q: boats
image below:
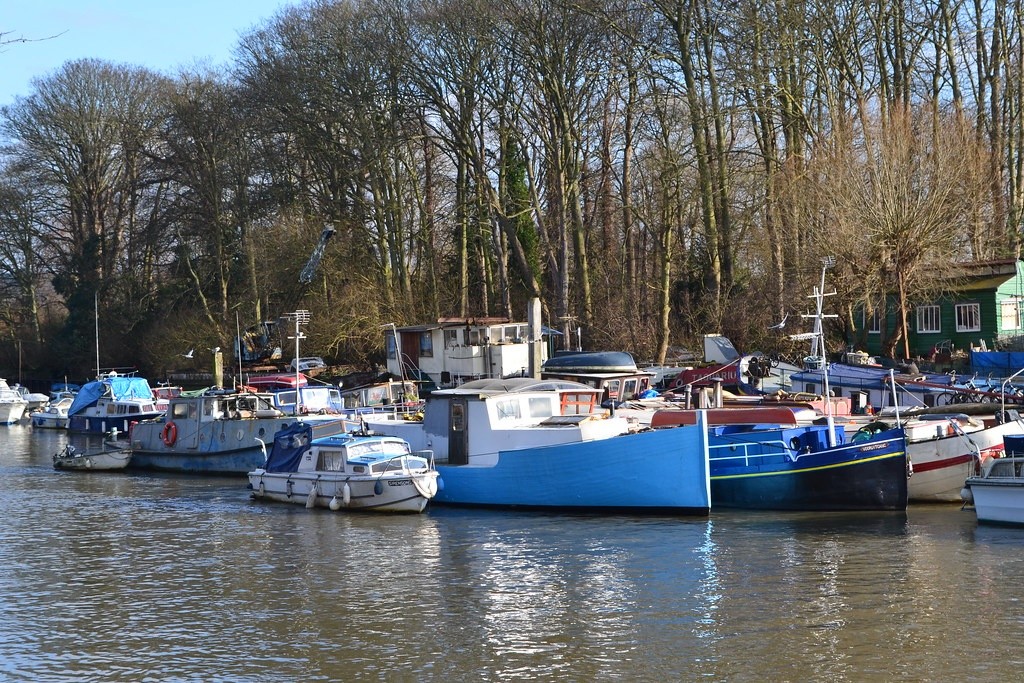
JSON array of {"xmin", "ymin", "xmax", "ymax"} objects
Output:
[{"xmin": 0, "ymin": 257, "xmax": 1023, "ymax": 531}]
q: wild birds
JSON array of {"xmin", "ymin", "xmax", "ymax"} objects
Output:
[
  {"xmin": 207, "ymin": 347, "xmax": 221, "ymax": 353},
  {"xmin": 180, "ymin": 350, "xmax": 195, "ymax": 358}
]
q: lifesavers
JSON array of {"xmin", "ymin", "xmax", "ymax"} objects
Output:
[{"xmin": 163, "ymin": 422, "xmax": 176, "ymax": 446}]
